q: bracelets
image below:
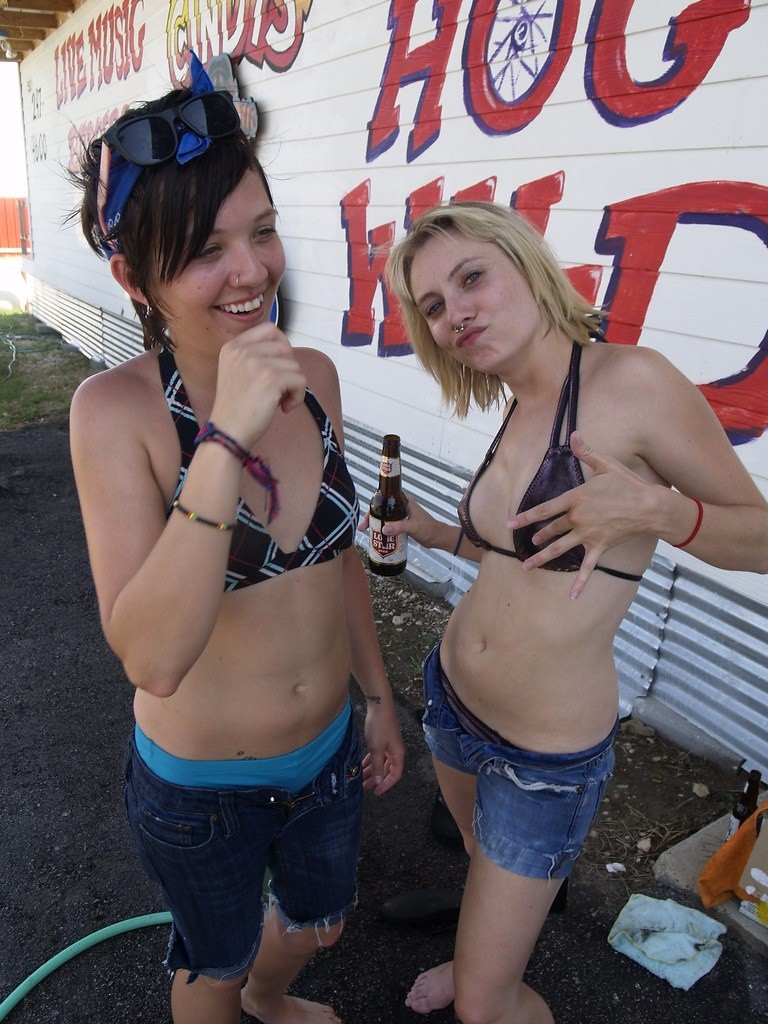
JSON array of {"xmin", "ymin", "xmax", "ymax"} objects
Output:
[
  {"xmin": 674, "ymin": 498, "xmax": 704, "ymax": 549},
  {"xmin": 173, "ymin": 502, "xmax": 237, "ymax": 530},
  {"xmin": 195, "ymin": 422, "xmax": 281, "ymax": 525}
]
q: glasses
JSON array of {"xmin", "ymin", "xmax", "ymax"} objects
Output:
[{"xmin": 98, "ymin": 89, "xmax": 241, "ymax": 252}]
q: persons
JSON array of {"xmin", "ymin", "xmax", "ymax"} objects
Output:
[
  {"xmin": 347, "ymin": 200, "xmax": 768, "ymax": 1024},
  {"xmin": 68, "ymin": 88, "xmax": 400, "ymax": 1024}
]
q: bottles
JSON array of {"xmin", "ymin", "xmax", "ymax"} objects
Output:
[
  {"xmin": 368, "ymin": 434, "xmax": 410, "ymax": 577},
  {"xmin": 725, "ymin": 770, "xmax": 761, "ymax": 842}
]
